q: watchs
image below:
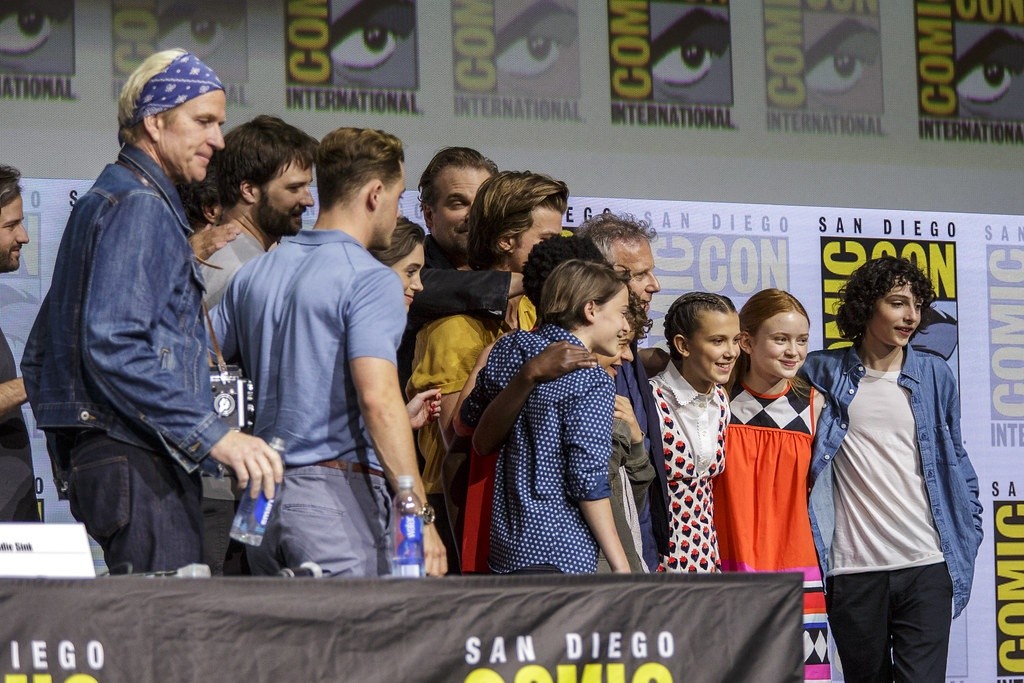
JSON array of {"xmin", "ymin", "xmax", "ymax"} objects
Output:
[{"xmin": 422, "ymin": 503, "xmax": 435, "ymax": 525}]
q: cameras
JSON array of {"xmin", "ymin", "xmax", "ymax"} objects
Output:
[{"xmin": 210, "ymin": 365, "xmax": 255, "ymax": 432}]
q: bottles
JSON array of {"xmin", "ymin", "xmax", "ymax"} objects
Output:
[
  {"xmin": 391, "ymin": 475, "xmax": 426, "ymax": 578},
  {"xmin": 230, "ymin": 436, "xmax": 285, "ymax": 545}
]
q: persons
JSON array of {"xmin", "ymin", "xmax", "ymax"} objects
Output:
[
  {"xmin": 178, "ymin": 116, "xmax": 670, "ymax": 578},
  {"xmin": 20, "ymin": 47, "xmax": 283, "ymax": 576},
  {"xmin": 648, "ymin": 291, "xmax": 741, "ymax": 574},
  {"xmin": 0, "ymin": 163, "xmax": 41, "ymax": 522},
  {"xmin": 795, "ymin": 257, "xmax": 984, "ymax": 683},
  {"xmin": 713, "ymin": 288, "xmax": 832, "ymax": 683}
]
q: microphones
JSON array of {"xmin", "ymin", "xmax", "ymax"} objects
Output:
[
  {"xmin": 278, "ymin": 561, "xmax": 322, "ymax": 577},
  {"xmin": 131, "ymin": 564, "xmax": 211, "ymax": 578}
]
